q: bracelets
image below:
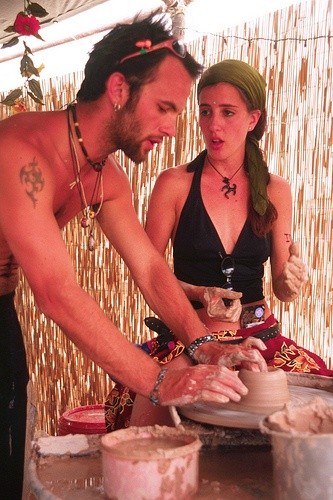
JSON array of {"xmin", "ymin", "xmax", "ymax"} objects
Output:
[
  {"xmin": 185, "ymin": 334, "xmax": 217, "ymax": 360},
  {"xmin": 149, "ymin": 367, "xmax": 169, "ymax": 406}
]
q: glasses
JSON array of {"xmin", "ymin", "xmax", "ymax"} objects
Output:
[
  {"xmin": 218, "ymin": 250, "xmax": 236, "ymax": 307},
  {"xmin": 117, "ymin": 35, "xmax": 188, "ymax": 65}
]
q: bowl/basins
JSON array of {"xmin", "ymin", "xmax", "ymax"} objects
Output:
[{"xmin": 99, "ymin": 425, "xmax": 202, "ymax": 500}]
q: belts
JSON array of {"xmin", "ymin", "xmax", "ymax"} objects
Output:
[{"xmin": 144, "ymin": 317, "xmax": 279, "ymax": 344}]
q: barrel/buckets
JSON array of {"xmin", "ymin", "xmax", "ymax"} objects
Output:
[
  {"xmin": 58, "ymin": 404, "xmax": 106, "ymax": 436},
  {"xmin": 259, "ymin": 403, "xmax": 332, "ymax": 500}
]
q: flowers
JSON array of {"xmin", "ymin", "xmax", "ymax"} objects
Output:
[{"xmin": 0, "ymin": 0, "xmax": 48, "ymax": 114}]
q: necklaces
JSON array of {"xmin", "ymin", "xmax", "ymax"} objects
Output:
[
  {"xmin": 66, "ymin": 102, "xmax": 109, "ymax": 253},
  {"xmin": 205, "ymin": 153, "xmax": 246, "ymax": 198}
]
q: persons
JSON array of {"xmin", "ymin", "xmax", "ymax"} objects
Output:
[
  {"xmin": 103, "ymin": 58, "xmax": 330, "ymax": 434},
  {"xmin": 0, "ymin": 9, "xmax": 205, "ymax": 500}
]
